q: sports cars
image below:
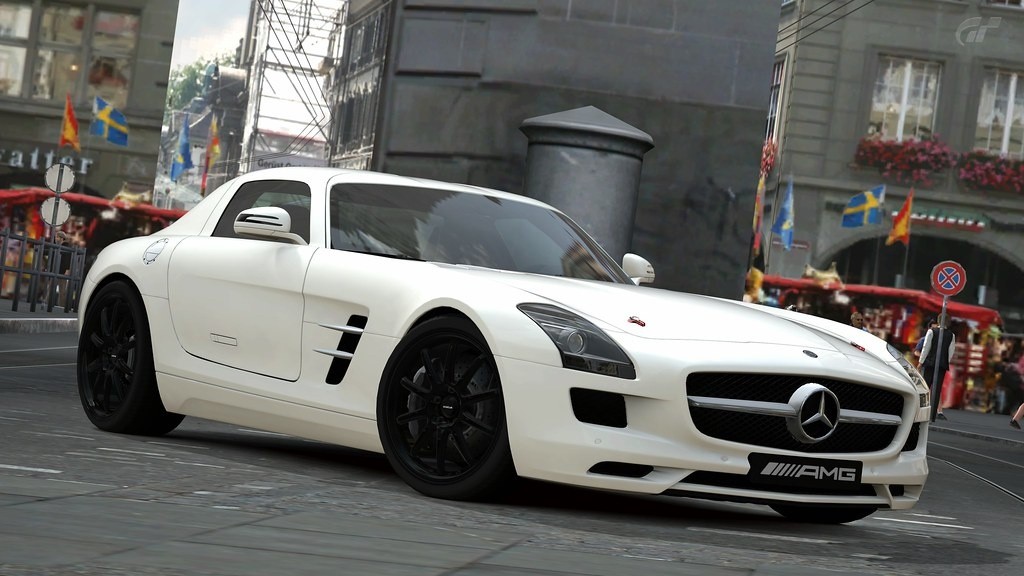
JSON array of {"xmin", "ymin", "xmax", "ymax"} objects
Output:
[{"xmin": 76, "ymin": 165, "xmax": 929, "ymax": 524}]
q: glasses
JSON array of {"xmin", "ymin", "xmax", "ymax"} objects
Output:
[{"xmin": 855, "ymin": 319, "xmax": 863, "ymax": 321}]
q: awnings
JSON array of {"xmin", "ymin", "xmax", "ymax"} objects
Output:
[{"xmin": 825, "ymin": 196, "xmax": 989, "ymax": 234}]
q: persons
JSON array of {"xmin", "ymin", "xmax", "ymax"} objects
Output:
[
  {"xmin": 851, "ymin": 311, "xmax": 868, "ymax": 332},
  {"xmin": 914, "ymin": 313, "xmax": 956, "ymax": 421},
  {"xmin": 47, "ymin": 231, "xmax": 72, "ymax": 308},
  {"xmin": 1001, "ymin": 340, "xmax": 1024, "ymax": 428}
]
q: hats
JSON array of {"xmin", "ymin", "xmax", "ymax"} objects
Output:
[{"xmin": 57, "ymin": 231, "xmax": 67, "ymax": 240}]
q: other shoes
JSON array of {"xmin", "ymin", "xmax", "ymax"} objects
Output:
[
  {"xmin": 938, "ymin": 413, "xmax": 948, "ymax": 420},
  {"xmin": 1008, "ymin": 420, "xmax": 1021, "ymax": 430}
]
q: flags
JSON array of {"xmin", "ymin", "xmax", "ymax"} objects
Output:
[
  {"xmin": 209, "ymin": 118, "xmax": 220, "ymax": 171},
  {"xmin": 771, "ymin": 176, "xmax": 795, "ymax": 250},
  {"xmin": 885, "ymin": 187, "xmax": 914, "ymax": 245},
  {"xmin": 170, "ymin": 113, "xmax": 195, "ymax": 182},
  {"xmin": 89, "ymin": 95, "xmax": 129, "ymax": 147},
  {"xmin": 59, "ymin": 92, "xmax": 81, "ymax": 152},
  {"xmin": 753, "ymin": 175, "xmax": 764, "ymax": 250},
  {"xmin": 842, "ymin": 185, "xmax": 884, "ymax": 228}
]
